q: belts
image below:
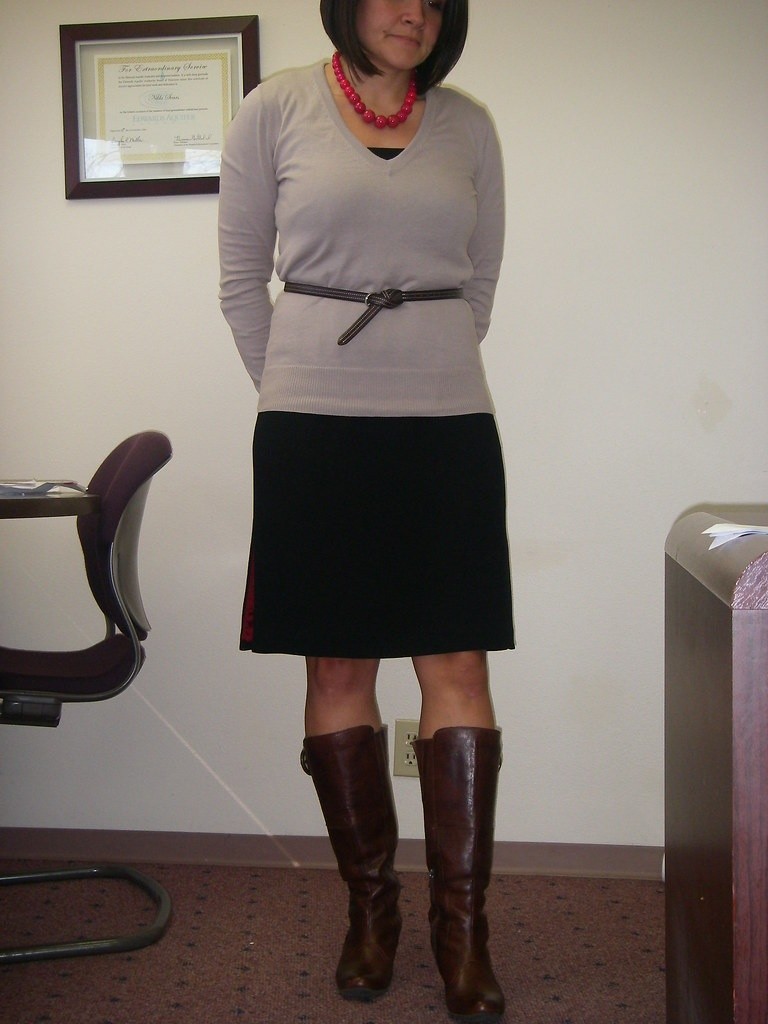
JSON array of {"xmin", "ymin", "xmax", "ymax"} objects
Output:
[{"xmin": 282, "ymin": 283, "xmax": 461, "ymax": 347}]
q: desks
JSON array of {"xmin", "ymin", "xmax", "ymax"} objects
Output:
[{"xmin": 0, "ymin": 478, "xmax": 102, "ymax": 519}]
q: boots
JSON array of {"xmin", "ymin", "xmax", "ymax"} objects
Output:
[
  {"xmin": 300, "ymin": 724, "xmax": 402, "ymax": 999},
  {"xmin": 411, "ymin": 725, "xmax": 505, "ymax": 1022}
]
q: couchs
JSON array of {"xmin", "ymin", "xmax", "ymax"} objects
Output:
[{"xmin": 660, "ymin": 502, "xmax": 768, "ymax": 1024}]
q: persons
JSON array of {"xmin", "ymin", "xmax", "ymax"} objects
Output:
[{"xmin": 219, "ymin": 0, "xmax": 515, "ymax": 1024}]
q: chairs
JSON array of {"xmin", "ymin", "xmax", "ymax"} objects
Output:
[{"xmin": 0, "ymin": 431, "xmax": 173, "ymax": 729}]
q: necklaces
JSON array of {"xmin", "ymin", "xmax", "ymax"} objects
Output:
[{"xmin": 332, "ymin": 52, "xmax": 417, "ymax": 128}]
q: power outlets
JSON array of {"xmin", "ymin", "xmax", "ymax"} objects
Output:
[{"xmin": 391, "ymin": 718, "xmax": 420, "ymax": 778}]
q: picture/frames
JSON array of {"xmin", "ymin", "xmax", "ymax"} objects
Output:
[{"xmin": 59, "ymin": 15, "xmax": 260, "ymax": 200}]
